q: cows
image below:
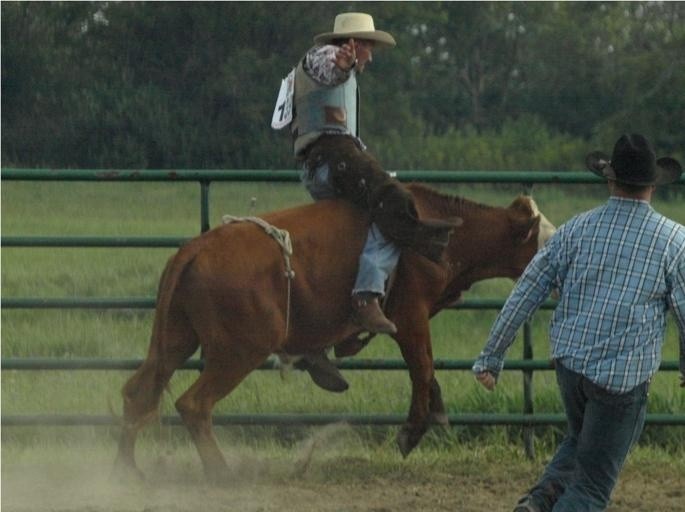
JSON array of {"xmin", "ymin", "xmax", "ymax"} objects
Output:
[{"xmin": 117, "ymin": 180, "xmax": 563, "ymax": 489}]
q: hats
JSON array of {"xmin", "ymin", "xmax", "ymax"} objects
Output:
[
  {"xmin": 313, "ymin": 10, "xmax": 397, "ymax": 50},
  {"xmin": 584, "ymin": 132, "xmax": 681, "ymax": 188}
]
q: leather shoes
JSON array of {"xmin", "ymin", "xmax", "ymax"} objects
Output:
[
  {"xmin": 304, "ymin": 351, "xmax": 350, "ymax": 394},
  {"xmin": 349, "ymin": 297, "xmax": 398, "ymax": 332}
]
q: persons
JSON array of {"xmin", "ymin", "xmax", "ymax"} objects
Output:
[
  {"xmin": 289, "ymin": 11, "xmax": 420, "ymax": 393},
  {"xmin": 468, "ymin": 132, "xmax": 684, "ymax": 512}
]
